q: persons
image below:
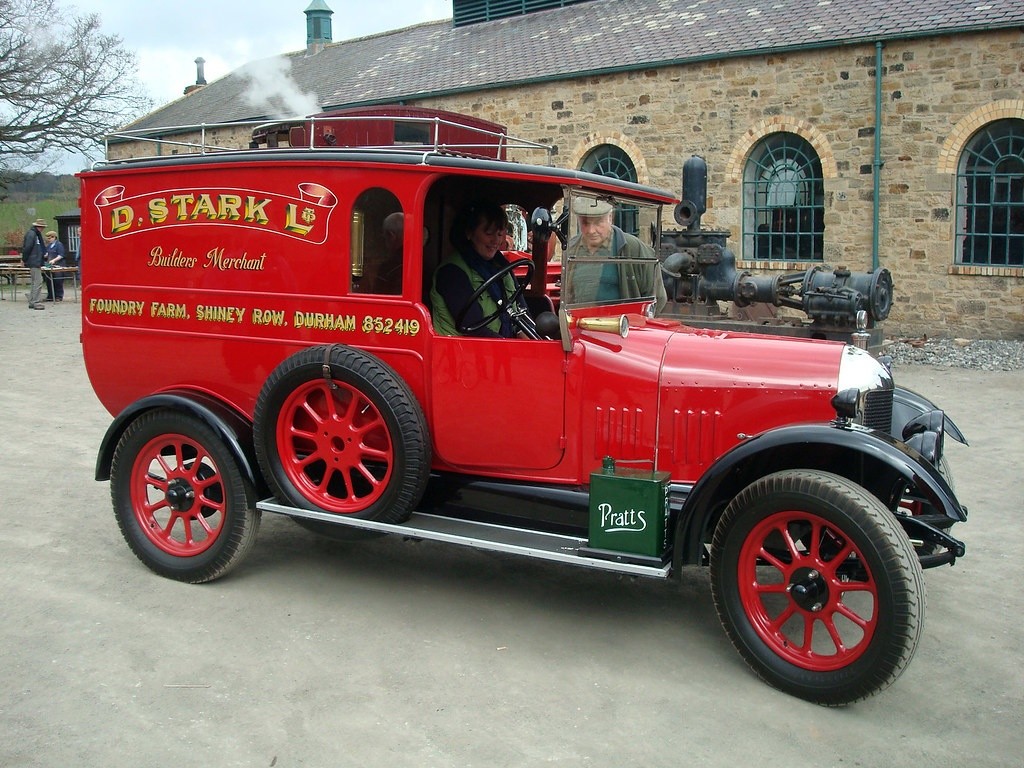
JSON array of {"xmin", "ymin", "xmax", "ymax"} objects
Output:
[
  {"xmin": 22, "ymin": 218, "xmax": 81, "ymax": 310},
  {"xmin": 561, "ymin": 198, "xmax": 668, "ymax": 314},
  {"xmin": 373, "ymin": 204, "xmax": 527, "ymax": 340}
]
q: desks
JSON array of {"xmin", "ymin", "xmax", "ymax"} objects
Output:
[{"xmin": 0, "ymin": 268, "xmax": 78, "ymax": 305}]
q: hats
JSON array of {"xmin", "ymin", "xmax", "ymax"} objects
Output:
[
  {"xmin": 32, "ymin": 218, "xmax": 48, "ymax": 227},
  {"xmin": 572, "ymin": 195, "xmax": 613, "ymax": 218},
  {"xmin": 46, "ymin": 231, "xmax": 58, "ymax": 237}
]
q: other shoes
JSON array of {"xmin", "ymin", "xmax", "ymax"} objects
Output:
[
  {"xmin": 44, "ymin": 298, "xmax": 63, "ymax": 302},
  {"xmin": 29, "ymin": 304, "xmax": 45, "ymax": 310}
]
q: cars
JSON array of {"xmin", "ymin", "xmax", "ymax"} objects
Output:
[{"xmin": 73, "ymin": 103, "xmax": 969, "ymax": 707}]
